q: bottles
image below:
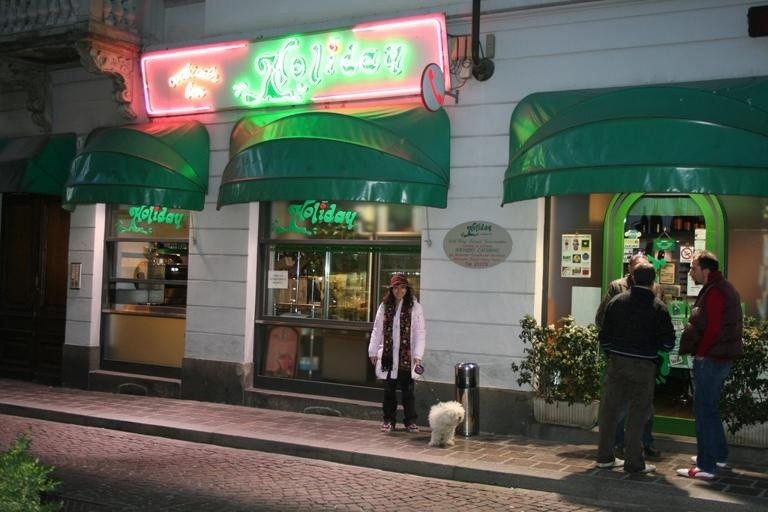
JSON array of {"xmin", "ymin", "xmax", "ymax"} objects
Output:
[{"xmin": 638, "ymin": 197, "xmax": 705, "ymax": 234}]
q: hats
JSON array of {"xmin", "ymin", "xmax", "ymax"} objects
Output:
[{"xmin": 386, "ymin": 273, "xmax": 414, "ymax": 289}]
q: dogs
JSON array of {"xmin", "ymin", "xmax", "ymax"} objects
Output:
[{"xmin": 427, "ymin": 400, "xmax": 466, "ymax": 447}]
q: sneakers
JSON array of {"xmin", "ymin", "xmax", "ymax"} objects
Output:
[
  {"xmin": 691, "ymin": 455, "xmax": 727, "ymax": 468},
  {"xmin": 597, "ymin": 442, "xmax": 661, "ymax": 459},
  {"xmin": 405, "ymin": 422, "xmax": 419, "ymax": 433},
  {"xmin": 381, "ymin": 422, "xmax": 395, "ymax": 432},
  {"xmin": 676, "ymin": 466, "xmax": 715, "ymax": 480},
  {"xmin": 595, "ymin": 457, "xmax": 626, "ymax": 468},
  {"xmin": 637, "ymin": 464, "xmax": 656, "ymax": 473}
]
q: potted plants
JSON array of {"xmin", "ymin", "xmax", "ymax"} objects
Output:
[
  {"xmin": 509, "ymin": 312, "xmax": 609, "ymax": 429},
  {"xmin": 718, "ymin": 310, "xmax": 768, "ymax": 450}
]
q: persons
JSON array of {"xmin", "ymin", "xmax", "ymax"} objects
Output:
[
  {"xmin": 367, "ymin": 275, "xmax": 425, "ymax": 433},
  {"xmin": 676, "ymin": 252, "xmax": 745, "ymax": 478},
  {"xmin": 597, "ymin": 263, "xmax": 676, "ymax": 473},
  {"xmin": 605, "ymin": 254, "xmax": 661, "ymax": 456}
]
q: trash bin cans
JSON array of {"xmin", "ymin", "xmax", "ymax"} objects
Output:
[{"xmin": 456, "ymin": 363, "xmax": 479, "ymax": 436}]
